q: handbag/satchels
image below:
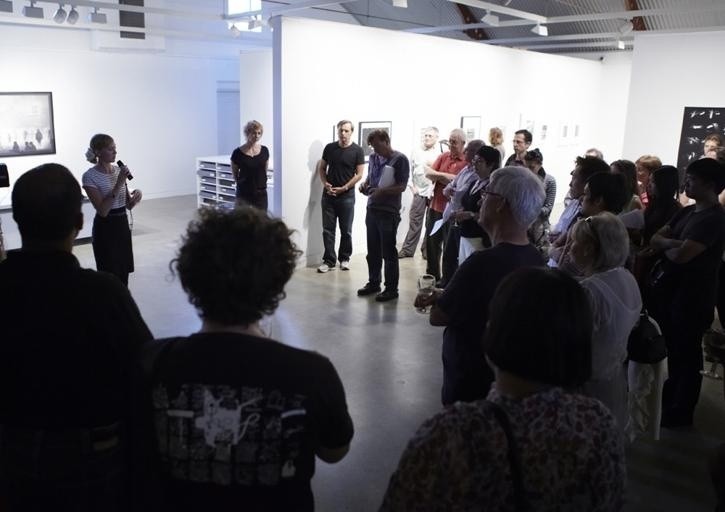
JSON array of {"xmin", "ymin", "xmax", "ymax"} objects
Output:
[{"xmin": 626, "ymin": 305, "xmax": 667, "ymax": 363}]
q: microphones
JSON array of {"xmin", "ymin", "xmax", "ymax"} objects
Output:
[{"xmin": 117, "ymin": 160, "xmax": 134, "ymax": 181}]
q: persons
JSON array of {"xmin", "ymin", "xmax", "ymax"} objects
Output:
[
  {"xmin": 421, "ymin": 130, "xmax": 471, "ymax": 279},
  {"xmin": 0, "ymin": 160, "xmax": 155, "ymax": 512},
  {"xmin": 428, "ymin": 166, "xmax": 546, "ymax": 414},
  {"xmin": 358, "ymin": 128, "xmax": 411, "ymax": 303},
  {"xmin": 558, "ymin": 212, "xmax": 644, "ymax": 465},
  {"xmin": 440, "ymin": 125, "xmax": 725, "ymax": 437},
  {"xmin": 108, "ymin": 207, "xmax": 358, "ymax": 511},
  {"xmin": 312, "ymin": 118, "xmax": 365, "ymax": 272},
  {"xmin": 396, "ymin": 125, "xmax": 451, "ymax": 261},
  {"xmin": 375, "ymin": 261, "xmax": 630, "ymax": 511},
  {"xmin": 230, "ymin": 122, "xmax": 270, "ymax": 217},
  {"xmin": 79, "ymin": 132, "xmax": 142, "ymax": 292}
]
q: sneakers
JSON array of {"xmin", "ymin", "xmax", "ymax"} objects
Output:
[
  {"xmin": 339, "ymin": 260, "xmax": 350, "ymax": 271},
  {"xmin": 316, "ymin": 263, "xmax": 336, "ymax": 273}
]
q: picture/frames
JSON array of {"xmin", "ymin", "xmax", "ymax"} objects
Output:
[
  {"xmin": 358, "ymin": 121, "xmax": 392, "ymax": 162},
  {"xmin": 461, "ymin": 115, "xmax": 480, "ymax": 146},
  {"xmin": 0, "ymin": 91, "xmax": 56, "ymax": 158}
]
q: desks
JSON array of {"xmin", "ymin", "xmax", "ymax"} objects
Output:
[{"xmin": 0, "ymin": 186, "xmax": 135, "ymax": 255}]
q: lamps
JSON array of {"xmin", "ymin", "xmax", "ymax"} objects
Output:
[
  {"xmin": 617, "ymin": 17, "xmax": 634, "ymax": 37},
  {"xmin": 0, "ymin": 0, "xmax": 107, "ymax": 25},
  {"xmin": 388, "ymin": 0, "xmax": 550, "ymax": 40},
  {"xmin": 223, "ymin": 0, "xmax": 264, "ymax": 35}
]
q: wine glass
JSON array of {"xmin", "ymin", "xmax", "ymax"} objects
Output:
[{"xmin": 415, "ymin": 272, "xmax": 436, "ymax": 316}]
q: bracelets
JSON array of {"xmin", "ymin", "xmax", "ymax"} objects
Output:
[
  {"xmin": 344, "ymin": 184, "xmax": 349, "ymax": 191},
  {"xmin": 114, "ymin": 182, "xmax": 124, "ymax": 188},
  {"xmin": 366, "ymin": 185, "xmax": 372, "ymax": 194}
]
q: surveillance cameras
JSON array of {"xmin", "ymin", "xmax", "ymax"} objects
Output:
[{"xmin": 599, "ymin": 56, "xmax": 604, "ymax": 61}]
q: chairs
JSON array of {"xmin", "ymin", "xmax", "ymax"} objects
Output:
[{"xmin": 0, "ymin": 162, "xmax": 10, "ymax": 187}]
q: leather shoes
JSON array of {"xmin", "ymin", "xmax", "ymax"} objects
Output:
[
  {"xmin": 397, "ymin": 250, "xmax": 413, "ymax": 258},
  {"xmin": 375, "ymin": 288, "xmax": 398, "ymax": 302},
  {"xmin": 357, "ymin": 284, "xmax": 381, "ymax": 296}
]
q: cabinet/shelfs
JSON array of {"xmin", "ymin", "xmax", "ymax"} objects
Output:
[{"xmin": 196, "ymin": 156, "xmax": 273, "ymax": 214}]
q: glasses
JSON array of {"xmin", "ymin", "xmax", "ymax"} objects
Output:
[
  {"xmin": 470, "ymin": 157, "xmax": 484, "ymax": 164},
  {"xmin": 479, "ymin": 188, "xmax": 502, "ymax": 201},
  {"xmin": 584, "ymin": 215, "xmax": 598, "ymax": 241}
]
